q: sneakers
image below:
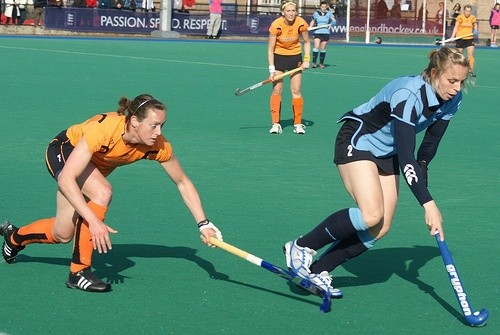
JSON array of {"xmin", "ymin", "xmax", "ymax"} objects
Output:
[
  {"xmin": 269, "ymin": 122, "xmax": 282, "ymax": 134},
  {"xmin": 66, "ymin": 266, "xmax": 111, "ymax": 292},
  {"xmin": 308, "ymin": 270, "xmax": 343, "ymax": 299},
  {"xmin": 293, "ymin": 124, "xmax": 306, "ymax": 134},
  {"xmin": 283, "ymin": 235, "xmax": 318, "ymax": 280},
  {"xmin": 0, "ymin": 220, "xmax": 25, "ymax": 264}
]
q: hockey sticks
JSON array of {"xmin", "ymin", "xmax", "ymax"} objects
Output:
[
  {"xmin": 435, "ymin": 32, "xmax": 475, "ymax": 46},
  {"xmin": 434, "ymin": 229, "xmax": 489, "ymax": 328},
  {"xmin": 308, "ymin": 23, "xmax": 331, "ymax": 30},
  {"xmin": 201, "ymin": 235, "xmax": 331, "ymax": 313},
  {"xmin": 234, "ymin": 66, "xmax": 302, "ymax": 97}
]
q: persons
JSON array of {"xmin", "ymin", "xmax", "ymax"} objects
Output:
[
  {"xmin": 269, "ymin": 1, "xmax": 311, "ymax": 134},
  {"xmin": 4, "ymin": 0, "xmax": 154, "ymax": 28},
  {"xmin": 282, "ymin": 47, "xmax": 470, "ymax": 297},
  {"xmin": 418, "ymin": 2, "xmax": 428, "ymax": 19},
  {"xmin": 173, "ymin": 0, "xmax": 196, "ymax": 13},
  {"xmin": 450, "ymin": 3, "xmax": 461, "ymax": 27},
  {"xmin": 450, "ymin": 5, "xmax": 477, "ymax": 77},
  {"xmin": 0, "ymin": 94, "xmax": 223, "ymax": 292},
  {"xmin": 206, "ymin": 0, "xmax": 222, "ymax": 39},
  {"xmin": 489, "ymin": 3, "xmax": 500, "ymax": 48},
  {"xmin": 376, "ymin": 0, "xmax": 401, "ymax": 19},
  {"xmin": 435, "ymin": 2, "xmax": 449, "ymax": 26},
  {"xmin": 307, "ymin": 1, "xmax": 336, "ymax": 68}
]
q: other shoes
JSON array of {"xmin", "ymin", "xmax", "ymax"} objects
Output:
[
  {"xmin": 207, "ymin": 36, "xmax": 211, "ymax": 38},
  {"xmin": 312, "ymin": 63, "xmax": 317, "ymax": 68},
  {"xmin": 469, "ymin": 70, "xmax": 477, "ymax": 77},
  {"xmin": 319, "ymin": 64, "xmax": 325, "ymax": 68},
  {"xmin": 212, "ymin": 36, "xmax": 218, "ymax": 39}
]
